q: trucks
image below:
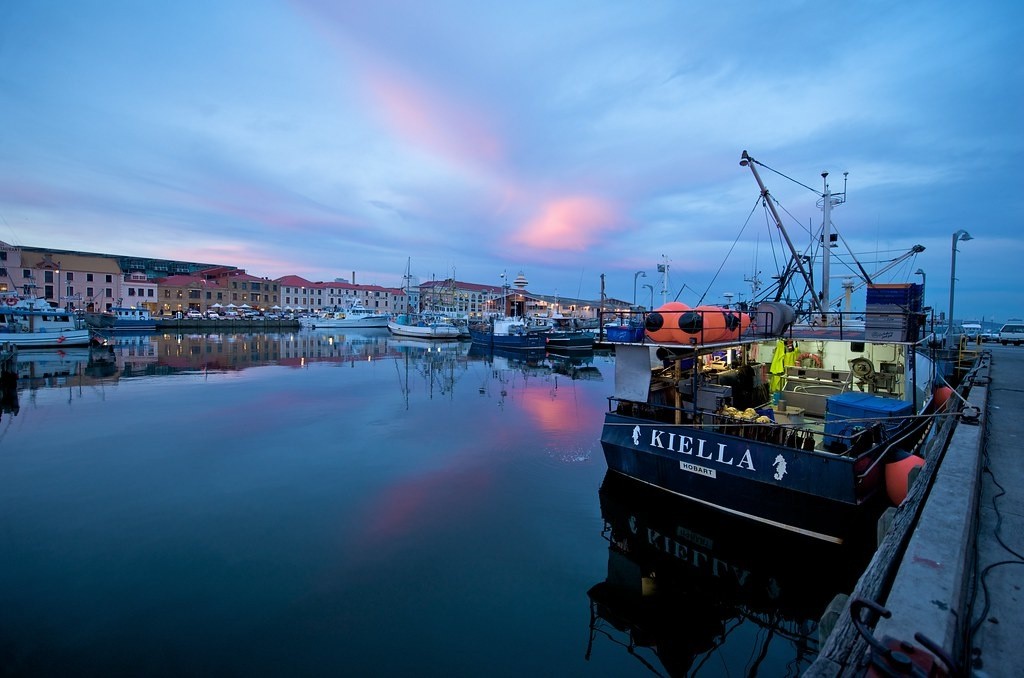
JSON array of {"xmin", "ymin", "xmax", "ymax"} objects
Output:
[{"xmin": 958, "ymin": 324, "xmax": 999, "ymax": 343}]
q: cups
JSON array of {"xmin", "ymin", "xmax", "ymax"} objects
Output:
[{"xmin": 778, "ymin": 400, "xmax": 786, "ymax": 411}]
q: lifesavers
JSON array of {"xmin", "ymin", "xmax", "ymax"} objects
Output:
[{"xmin": 795, "ymin": 352, "xmax": 822, "ymax": 368}]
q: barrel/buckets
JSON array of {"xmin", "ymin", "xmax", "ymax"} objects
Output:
[{"xmin": 645, "ymin": 301, "xmax": 795, "ymax": 344}]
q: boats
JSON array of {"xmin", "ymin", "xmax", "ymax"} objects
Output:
[
  {"xmin": 0, "ymin": 342, "xmax": 91, "ymax": 383},
  {"xmin": 311, "ymin": 256, "xmax": 646, "ymax": 355},
  {"xmin": 600, "ymin": 148, "xmax": 979, "ymax": 591},
  {"xmin": 0, "ymin": 246, "xmax": 107, "ymax": 349}
]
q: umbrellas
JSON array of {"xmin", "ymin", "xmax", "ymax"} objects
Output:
[{"xmin": 210, "ymin": 303, "xmax": 304, "ymax": 310}]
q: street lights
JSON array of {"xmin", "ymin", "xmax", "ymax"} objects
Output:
[
  {"xmin": 914, "ymin": 269, "xmax": 926, "ymax": 312},
  {"xmin": 634, "ymin": 271, "xmax": 647, "ymax": 305},
  {"xmin": 945, "ymin": 228, "xmax": 975, "ymax": 351},
  {"xmin": 641, "ymin": 284, "xmax": 653, "ymax": 311}
]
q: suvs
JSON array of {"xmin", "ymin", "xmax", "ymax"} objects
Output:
[
  {"xmin": 926, "ymin": 324, "xmax": 967, "ymax": 350},
  {"xmin": 999, "ymin": 322, "xmax": 1024, "ymax": 346}
]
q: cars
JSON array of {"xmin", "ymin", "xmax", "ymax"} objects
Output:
[
  {"xmin": 243, "ymin": 310, "xmax": 254, "ymax": 317},
  {"xmin": 240, "ymin": 307, "xmax": 309, "ymax": 320},
  {"xmin": 224, "ymin": 310, "xmax": 239, "ymax": 317},
  {"xmin": 203, "ymin": 310, "xmax": 219, "ymax": 321},
  {"xmin": 186, "ymin": 310, "xmax": 202, "ymax": 320}
]
